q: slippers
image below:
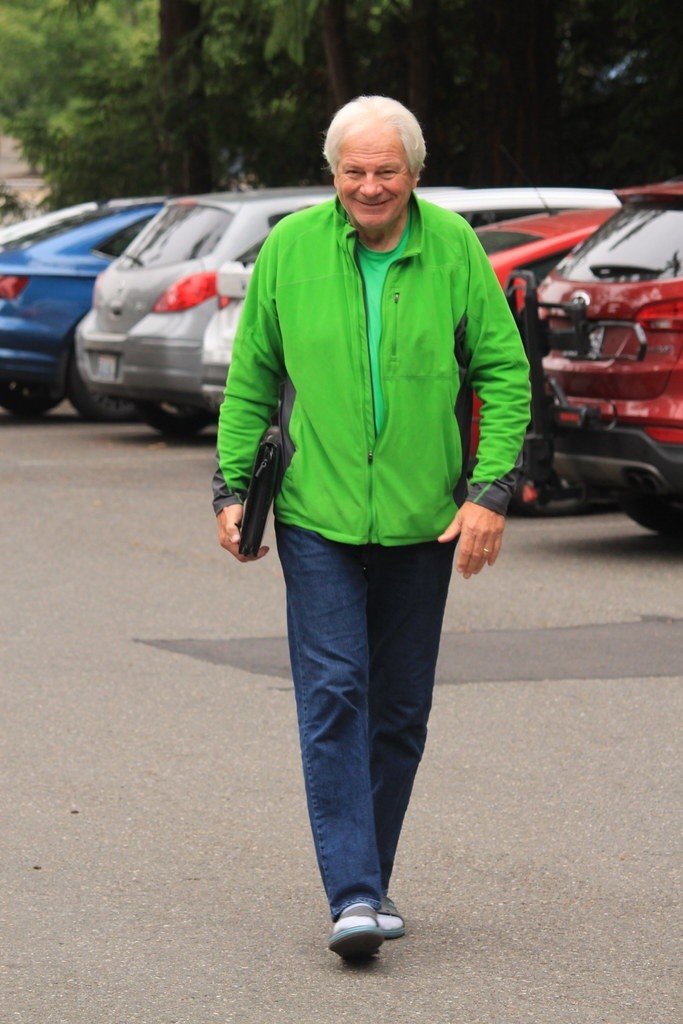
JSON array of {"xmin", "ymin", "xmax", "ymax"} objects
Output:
[
  {"xmin": 378, "ymin": 896, "xmax": 405, "ymax": 938},
  {"xmin": 327, "ymin": 907, "xmax": 383, "ymax": 960}
]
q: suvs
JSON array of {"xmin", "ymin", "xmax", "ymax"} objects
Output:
[
  {"xmin": 537, "ymin": 178, "xmax": 683, "ymax": 539},
  {"xmin": 202, "ymin": 207, "xmax": 277, "ymax": 406},
  {"xmin": 74, "ymin": 187, "xmax": 338, "ymax": 441}
]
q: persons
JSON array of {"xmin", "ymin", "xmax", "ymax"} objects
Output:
[{"xmin": 211, "ymin": 96, "xmax": 532, "ymax": 964}]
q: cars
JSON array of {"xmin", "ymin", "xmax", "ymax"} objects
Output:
[
  {"xmin": 0, "ymin": 199, "xmax": 162, "ymax": 425},
  {"xmin": 460, "ymin": 208, "xmax": 621, "ymax": 461}
]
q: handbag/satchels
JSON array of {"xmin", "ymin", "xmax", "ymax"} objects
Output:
[{"xmin": 237, "ymin": 426, "xmax": 282, "ymax": 557}]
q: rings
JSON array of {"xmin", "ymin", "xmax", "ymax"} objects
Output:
[{"xmin": 483, "ymin": 548, "xmax": 492, "ymax": 553}]
